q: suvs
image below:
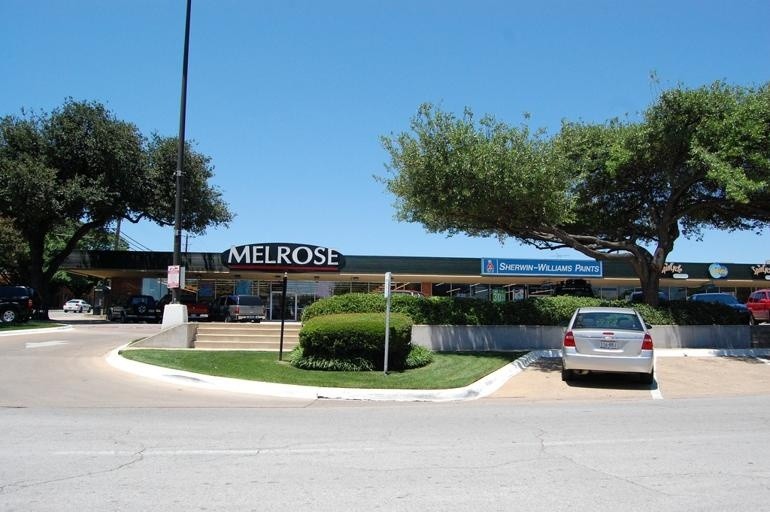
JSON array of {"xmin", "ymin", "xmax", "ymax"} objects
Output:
[{"xmin": 0, "ymin": 286, "xmax": 41, "ymax": 323}]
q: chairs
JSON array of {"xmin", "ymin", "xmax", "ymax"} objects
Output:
[
  {"xmin": 616, "ymin": 320, "xmax": 634, "ymax": 329},
  {"xmin": 582, "ymin": 318, "xmax": 595, "ymax": 327}
]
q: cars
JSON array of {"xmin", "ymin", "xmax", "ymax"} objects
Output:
[
  {"xmin": 562, "ymin": 307, "xmax": 653, "ymax": 384},
  {"xmin": 63, "ymin": 299, "xmax": 91, "ymax": 313},
  {"xmin": 688, "ymin": 290, "xmax": 770, "ymax": 325},
  {"xmin": 108, "ymin": 295, "xmax": 265, "ymax": 323},
  {"xmin": 391, "ymin": 290, "xmax": 424, "ymax": 296},
  {"xmin": 627, "ymin": 292, "xmax": 664, "ymax": 301}
]
